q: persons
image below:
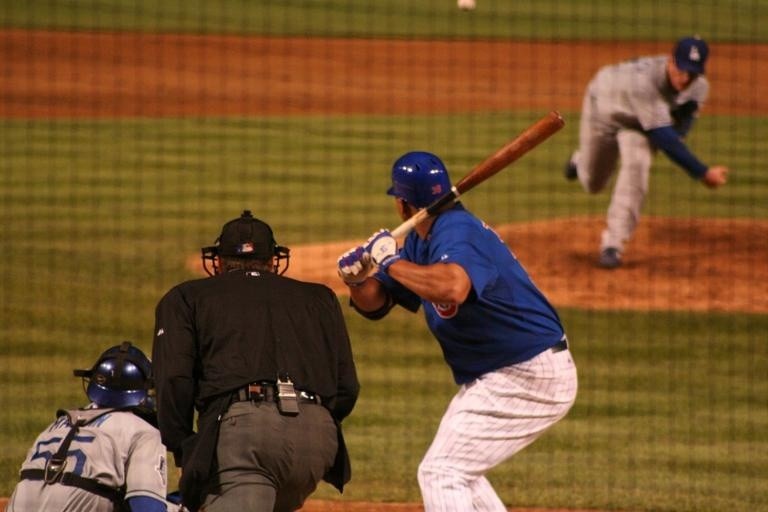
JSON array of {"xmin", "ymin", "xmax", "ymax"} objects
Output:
[
  {"xmin": 337, "ymin": 150, "xmax": 578, "ymax": 512},
  {"xmin": 151, "ymin": 208, "xmax": 361, "ymax": 512},
  {"xmin": 4, "ymin": 342, "xmax": 189, "ymax": 511},
  {"xmin": 566, "ymin": 34, "xmax": 730, "ymax": 271}
]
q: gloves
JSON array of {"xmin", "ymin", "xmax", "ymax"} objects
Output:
[
  {"xmin": 337, "ymin": 245, "xmax": 370, "ymax": 288},
  {"xmin": 363, "ymin": 227, "xmax": 401, "ymax": 272}
]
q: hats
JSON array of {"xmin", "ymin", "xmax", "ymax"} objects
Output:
[
  {"xmin": 386, "ymin": 151, "xmax": 452, "ymax": 206},
  {"xmin": 87, "ymin": 343, "xmax": 153, "ymax": 408},
  {"xmin": 217, "ymin": 215, "xmax": 276, "ymax": 258},
  {"xmin": 674, "ymin": 36, "xmax": 710, "ymax": 74}
]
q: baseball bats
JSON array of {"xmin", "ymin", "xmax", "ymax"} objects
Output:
[{"xmin": 390, "ymin": 109, "xmax": 565, "ymax": 239}]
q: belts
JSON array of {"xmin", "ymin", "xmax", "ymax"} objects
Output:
[
  {"xmin": 550, "ymin": 339, "xmax": 568, "ymax": 354},
  {"xmin": 229, "ymin": 386, "xmax": 327, "ymax": 407}
]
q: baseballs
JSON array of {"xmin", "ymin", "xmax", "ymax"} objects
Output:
[{"xmin": 456, "ymin": 1, "xmax": 475, "ymax": 12}]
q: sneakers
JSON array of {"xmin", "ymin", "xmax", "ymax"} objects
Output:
[
  {"xmin": 566, "ymin": 157, "xmax": 578, "ymax": 181},
  {"xmin": 599, "ymin": 247, "xmax": 622, "ymax": 268}
]
